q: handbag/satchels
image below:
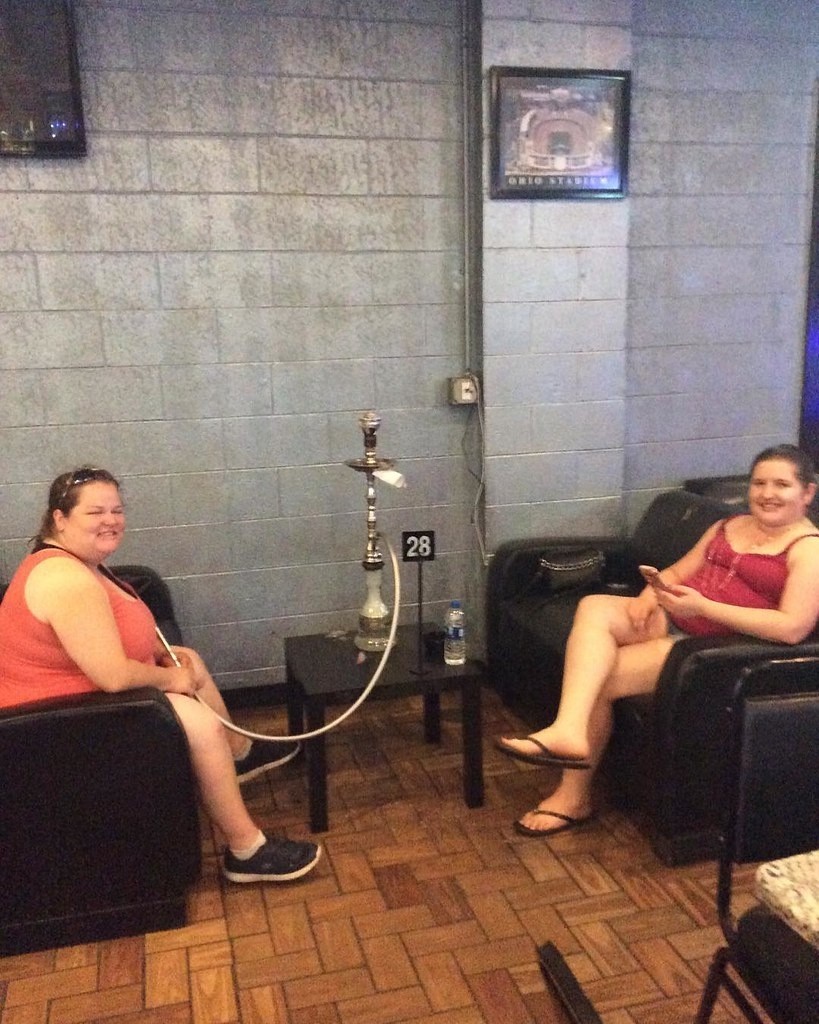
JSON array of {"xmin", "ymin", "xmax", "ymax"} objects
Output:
[{"xmin": 538, "ymin": 547, "xmax": 606, "ymax": 592}]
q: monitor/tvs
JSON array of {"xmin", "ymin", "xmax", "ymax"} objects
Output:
[{"xmin": 0, "ymin": 0, "xmax": 87, "ymax": 158}]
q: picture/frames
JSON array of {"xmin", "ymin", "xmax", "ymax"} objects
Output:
[{"xmin": 489, "ymin": 65, "xmax": 632, "ymax": 200}]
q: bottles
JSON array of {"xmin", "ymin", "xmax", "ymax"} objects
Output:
[{"xmin": 442, "ymin": 601, "xmax": 467, "ymax": 664}]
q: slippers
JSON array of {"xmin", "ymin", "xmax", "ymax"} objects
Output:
[
  {"xmin": 495, "ymin": 736, "xmax": 590, "ymax": 770},
  {"xmin": 513, "ymin": 808, "xmax": 598, "ymax": 837}
]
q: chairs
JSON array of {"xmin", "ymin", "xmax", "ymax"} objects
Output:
[{"xmin": 695, "ymin": 656, "xmax": 819, "ymax": 1024}]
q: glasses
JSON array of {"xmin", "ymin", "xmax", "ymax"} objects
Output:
[{"xmin": 62, "ymin": 468, "xmax": 96, "ymax": 503}]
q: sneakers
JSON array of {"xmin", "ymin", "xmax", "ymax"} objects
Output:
[
  {"xmin": 222, "ymin": 829, "xmax": 321, "ymax": 883},
  {"xmin": 234, "ymin": 740, "xmax": 301, "ymax": 784}
]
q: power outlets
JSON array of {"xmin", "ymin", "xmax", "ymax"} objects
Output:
[{"xmin": 448, "ymin": 376, "xmax": 483, "ymax": 406}]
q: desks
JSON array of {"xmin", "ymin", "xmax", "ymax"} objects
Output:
[{"xmin": 282, "ymin": 620, "xmax": 488, "ymax": 836}]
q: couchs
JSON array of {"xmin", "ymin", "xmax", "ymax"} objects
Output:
[
  {"xmin": 488, "ymin": 492, "xmax": 819, "ymax": 864},
  {"xmin": 0, "ymin": 565, "xmax": 203, "ymax": 958}
]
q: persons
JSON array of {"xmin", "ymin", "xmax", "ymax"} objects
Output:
[
  {"xmin": 495, "ymin": 443, "xmax": 819, "ymax": 836},
  {"xmin": 0, "ymin": 469, "xmax": 321, "ymax": 881}
]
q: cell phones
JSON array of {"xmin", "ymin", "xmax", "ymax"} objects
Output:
[{"xmin": 638, "ymin": 563, "xmax": 667, "ymax": 597}]
